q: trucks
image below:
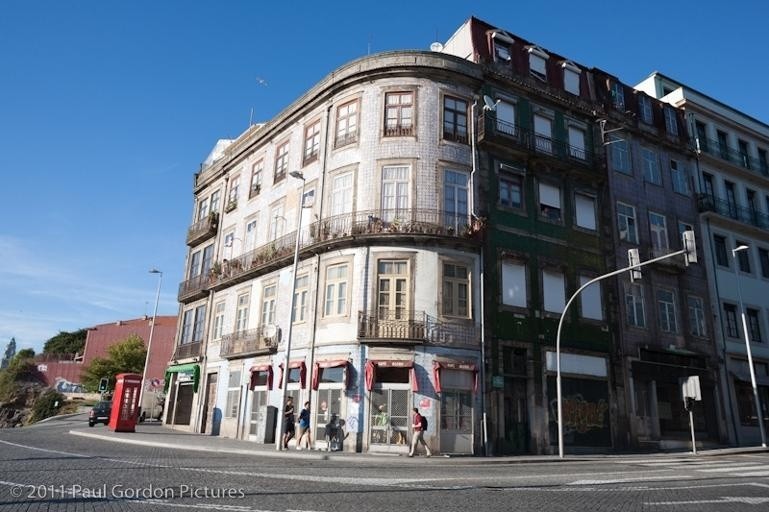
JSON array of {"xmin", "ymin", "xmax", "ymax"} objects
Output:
[{"xmin": 139, "ymin": 390, "xmax": 164, "ymax": 423}]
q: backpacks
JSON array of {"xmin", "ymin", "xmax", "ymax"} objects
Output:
[{"xmin": 416, "ymin": 413, "xmax": 427, "ymax": 431}]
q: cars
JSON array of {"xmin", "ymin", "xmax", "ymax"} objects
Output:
[{"xmin": 88, "ymin": 400, "xmax": 114, "ymax": 427}]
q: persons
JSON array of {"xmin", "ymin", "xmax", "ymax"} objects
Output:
[
  {"xmin": 407, "ymin": 408, "xmax": 433, "ymax": 458},
  {"xmin": 281, "ymin": 396, "xmax": 295, "ymax": 449},
  {"xmin": 296, "ymin": 402, "xmax": 314, "ymax": 451}
]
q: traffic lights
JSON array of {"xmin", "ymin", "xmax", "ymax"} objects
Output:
[{"xmin": 97, "ymin": 375, "xmax": 110, "ymax": 393}]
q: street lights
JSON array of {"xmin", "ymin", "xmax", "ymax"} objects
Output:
[
  {"xmin": 274, "ymin": 167, "xmax": 307, "ymax": 452},
  {"xmin": 138, "ymin": 267, "xmax": 164, "ymax": 408},
  {"xmin": 730, "ymin": 244, "xmax": 766, "ymax": 451}
]
